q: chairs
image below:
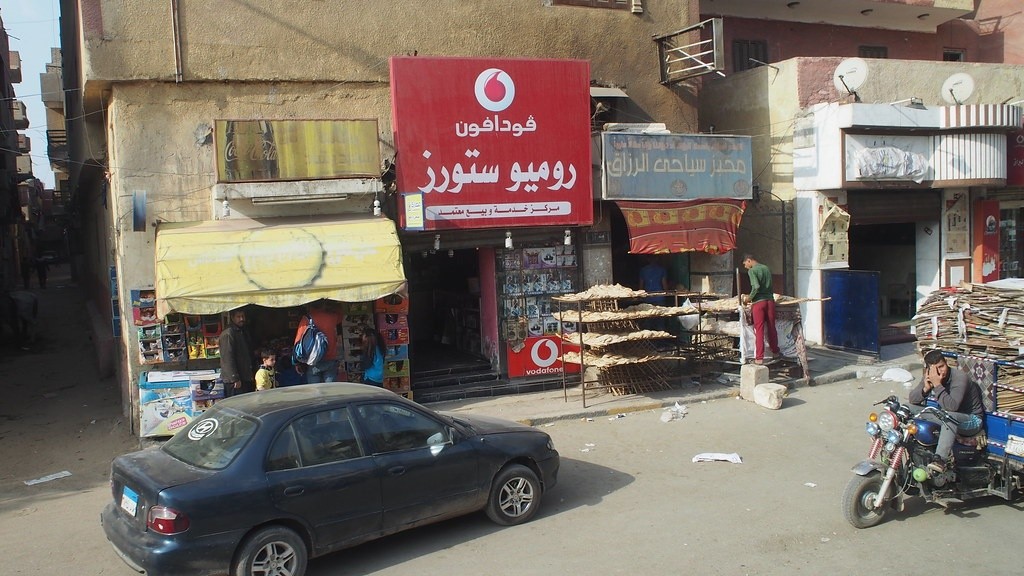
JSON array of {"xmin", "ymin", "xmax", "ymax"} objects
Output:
[
  {"xmin": 889, "ymin": 272, "xmax": 916, "ymax": 319},
  {"xmin": 287, "ymin": 413, "xmax": 359, "ymax": 467}
]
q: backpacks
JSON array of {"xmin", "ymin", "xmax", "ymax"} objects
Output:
[{"xmin": 291, "ymin": 311, "xmax": 329, "ymax": 366}]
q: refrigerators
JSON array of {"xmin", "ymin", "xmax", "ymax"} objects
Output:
[{"xmin": 140, "ymin": 370, "xmax": 224, "ymax": 437}]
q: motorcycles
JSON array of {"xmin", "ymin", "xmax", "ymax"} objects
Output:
[{"xmin": 842, "ymin": 348, "xmax": 1024, "ymax": 530}]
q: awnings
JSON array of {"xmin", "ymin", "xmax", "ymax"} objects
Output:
[{"xmin": 154, "ymin": 213, "xmax": 409, "ymax": 320}]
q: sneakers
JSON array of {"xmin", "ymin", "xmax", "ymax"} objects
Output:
[{"xmin": 927, "ymin": 453, "xmax": 946, "ymax": 474}]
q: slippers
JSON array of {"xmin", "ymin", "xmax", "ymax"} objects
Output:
[
  {"xmin": 773, "ymin": 353, "xmax": 783, "ymax": 360},
  {"xmin": 748, "ymin": 359, "xmax": 763, "ymax": 365}
]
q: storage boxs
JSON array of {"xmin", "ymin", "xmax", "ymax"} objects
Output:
[
  {"xmin": 335, "ymin": 292, "xmax": 413, "ymax": 402},
  {"xmin": 502, "ymin": 296, "xmax": 576, "ymax": 342},
  {"xmin": 131, "ymin": 289, "xmax": 224, "ymax": 364},
  {"xmin": 498, "ymin": 245, "xmax": 578, "ymax": 270},
  {"xmin": 502, "ymin": 274, "xmax": 572, "ymax": 293}
]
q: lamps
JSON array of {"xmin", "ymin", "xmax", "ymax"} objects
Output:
[
  {"xmin": 564, "ymin": 228, "xmax": 571, "ymax": 246},
  {"xmin": 505, "ymin": 230, "xmax": 513, "ymax": 248},
  {"xmin": 373, "ymin": 177, "xmax": 381, "ymax": 216},
  {"xmin": 223, "ymin": 196, "xmax": 230, "ymax": 218},
  {"xmin": 433, "ymin": 235, "xmax": 441, "ymax": 250},
  {"xmin": 891, "ymin": 96, "xmax": 927, "ymax": 110},
  {"xmin": 448, "ymin": 249, "xmax": 454, "ymax": 258}
]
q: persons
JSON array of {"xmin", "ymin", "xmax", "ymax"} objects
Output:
[
  {"xmin": 0, "ymin": 291, "xmax": 38, "ymax": 350},
  {"xmin": 360, "ymin": 327, "xmax": 386, "ymax": 387},
  {"xmin": 740, "ymin": 254, "xmax": 783, "ymax": 365},
  {"xmin": 903, "ymin": 349, "xmax": 985, "ymax": 474},
  {"xmin": 638, "ymin": 254, "xmax": 667, "ymax": 342},
  {"xmin": 218, "ymin": 309, "xmax": 256, "ymax": 397},
  {"xmin": 292, "ymin": 300, "xmax": 345, "ymax": 384},
  {"xmin": 255, "ymin": 349, "xmax": 276, "ymax": 391},
  {"xmin": 21, "ymin": 256, "xmax": 50, "ymax": 289},
  {"xmin": 196, "ymin": 385, "xmax": 205, "ymax": 397}
]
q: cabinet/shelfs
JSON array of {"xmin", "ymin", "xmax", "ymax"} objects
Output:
[
  {"xmin": 432, "ymin": 288, "xmax": 483, "ymax": 359},
  {"xmin": 550, "ymin": 292, "xmax": 832, "ymax": 408},
  {"xmin": 497, "ymin": 242, "xmax": 584, "ymax": 383}
]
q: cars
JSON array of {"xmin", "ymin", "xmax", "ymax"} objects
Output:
[{"xmin": 101, "ymin": 383, "xmax": 561, "ymax": 576}]
